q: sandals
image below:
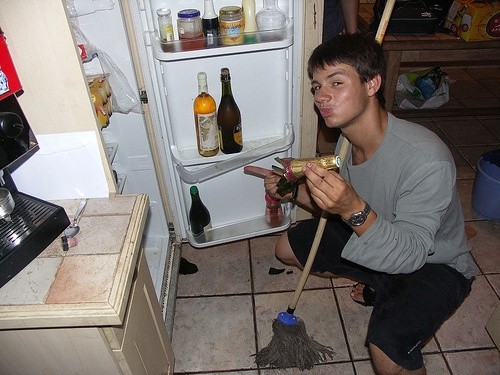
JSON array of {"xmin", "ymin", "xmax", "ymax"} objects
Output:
[{"xmin": 350, "ymin": 282, "xmax": 377, "ymax": 306}]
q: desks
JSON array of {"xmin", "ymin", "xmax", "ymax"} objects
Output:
[{"xmin": 356, "ymin": 0, "xmax": 500, "ymax": 119}]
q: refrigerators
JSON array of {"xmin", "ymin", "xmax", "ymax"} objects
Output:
[{"xmin": 0, "ymin": 1, "xmax": 325, "ymax": 338}]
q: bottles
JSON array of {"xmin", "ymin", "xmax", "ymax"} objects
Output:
[
  {"xmin": 157, "ymin": 1, "xmax": 218, "ymax": 52},
  {"xmin": 266, "ymin": 194, "xmax": 283, "ymax": 226},
  {"xmin": 189, "ymin": 185, "xmax": 214, "ymax": 243},
  {"xmin": 217, "ymin": 68, "xmax": 244, "ymax": 154},
  {"xmin": 256, "ymin": 0, "xmax": 287, "ymax": 44},
  {"xmin": 192, "ymin": 73, "xmax": 220, "ymax": 156},
  {"xmin": 86, "ymin": 72, "xmax": 112, "ymax": 129},
  {"xmin": 242, "ymin": 0, "xmax": 256, "ymax": 44}
]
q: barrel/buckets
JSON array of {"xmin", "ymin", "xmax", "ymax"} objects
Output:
[{"xmin": 473, "ymin": 155, "xmax": 500, "ymax": 222}]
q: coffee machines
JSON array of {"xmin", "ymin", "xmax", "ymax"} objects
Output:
[{"xmin": 0, "ymin": 94, "xmax": 72, "ymax": 288}]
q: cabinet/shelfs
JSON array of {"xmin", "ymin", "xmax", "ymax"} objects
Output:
[{"xmin": 0, "ymin": 193, "xmax": 175, "ymax": 375}]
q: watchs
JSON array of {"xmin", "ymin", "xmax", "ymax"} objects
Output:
[{"xmin": 342, "ymin": 201, "xmax": 372, "ymax": 226}]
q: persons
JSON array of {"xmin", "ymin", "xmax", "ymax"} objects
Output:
[
  {"xmin": 242, "ymin": 33, "xmax": 476, "ymax": 375},
  {"xmin": 323, "ymin": 0, "xmax": 360, "ymax": 41}
]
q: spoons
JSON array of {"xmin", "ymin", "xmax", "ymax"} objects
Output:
[{"xmin": 65, "ymin": 198, "xmax": 87, "ymax": 237}]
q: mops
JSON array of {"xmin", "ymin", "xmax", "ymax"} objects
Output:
[{"xmin": 254, "ymin": 0, "xmax": 399, "ymax": 369}]
